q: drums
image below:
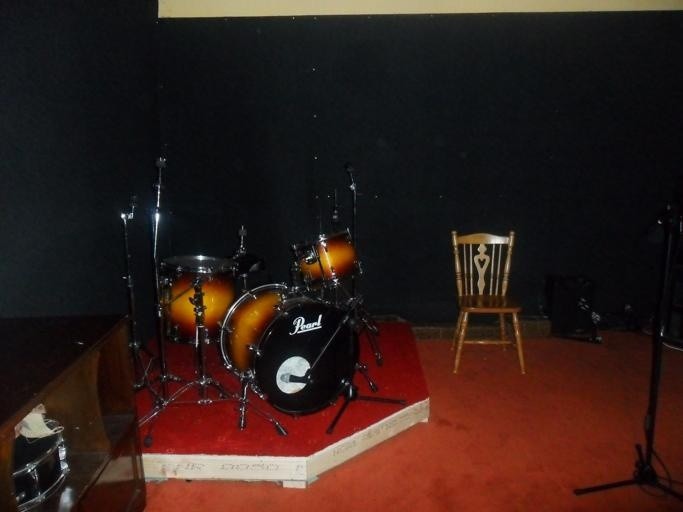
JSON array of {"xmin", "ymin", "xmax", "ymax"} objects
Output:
[
  {"xmin": 11, "ymin": 420, "xmax": 70, "ymax": 509},
  {"xmin": 292, "ymin": 227, "xmax": 364, "ymax": 293},
  {"xmin": 155, "ymin": 253, "xmax": 239, "ymax": 343},
  {"xmin": 217, "ymin": 281, "xmax": 360, "ymax": 418}
]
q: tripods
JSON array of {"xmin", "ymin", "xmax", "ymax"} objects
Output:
[
  {"xmin": 573, "ymin": 224, "xmax": 683, "ymax": 501},
  {"xmin": 325, "ymin": 193, "xmax": 408, "ymax": 436}
]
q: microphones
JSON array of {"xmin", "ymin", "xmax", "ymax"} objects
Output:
[
  {"xmin": 344, "ymin": 161, "xmax": 356, "ymax": 191},
  {"xmin": 331, "ymin": 188, "xmax": 340, "ymax": 223},
  {"xmin": 280, "ymin": 374, "xmax": 314, "ymax": 385}
]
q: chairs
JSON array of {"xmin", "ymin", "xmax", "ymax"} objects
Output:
[{"xmin": 447, "ymin": 228, "xmax": 526, "ymax": 374}]
